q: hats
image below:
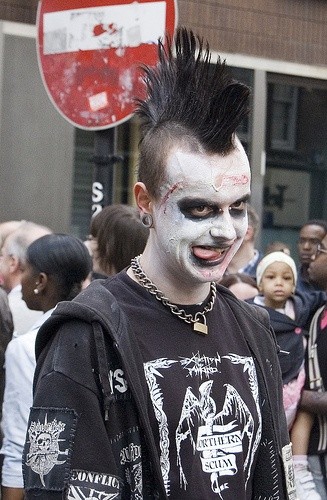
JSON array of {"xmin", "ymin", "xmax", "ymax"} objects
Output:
[{"xmin": 256, "ymin": 251, "xmax": 297, "ymax": 286}]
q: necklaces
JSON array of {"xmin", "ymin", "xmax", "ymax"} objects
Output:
[{"xmin": 130, "ymin": 254, "xmax": 216, "ymax": 336}]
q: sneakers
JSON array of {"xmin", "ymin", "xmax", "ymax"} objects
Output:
[{"xmin": 292, "ymin": 454, "xmax": 320, "ymax": 500}]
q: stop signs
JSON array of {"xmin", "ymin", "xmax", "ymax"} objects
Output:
[{"xmin": 35, "ymin": 0, "xmax": 179, "ymax": 131}]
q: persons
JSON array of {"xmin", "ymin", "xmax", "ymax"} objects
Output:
[
  {"xmin": 0, "ymin": 204, "xmax": 325, "ymax": 500},
  {"xmin": 21, "ymin": 26, "xmax": 298, "ymax": 500}
]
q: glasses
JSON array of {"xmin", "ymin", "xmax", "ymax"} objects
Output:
[{"xmin": 315, "ymin": 243, "xmax": 327, "ymax": 257}]
